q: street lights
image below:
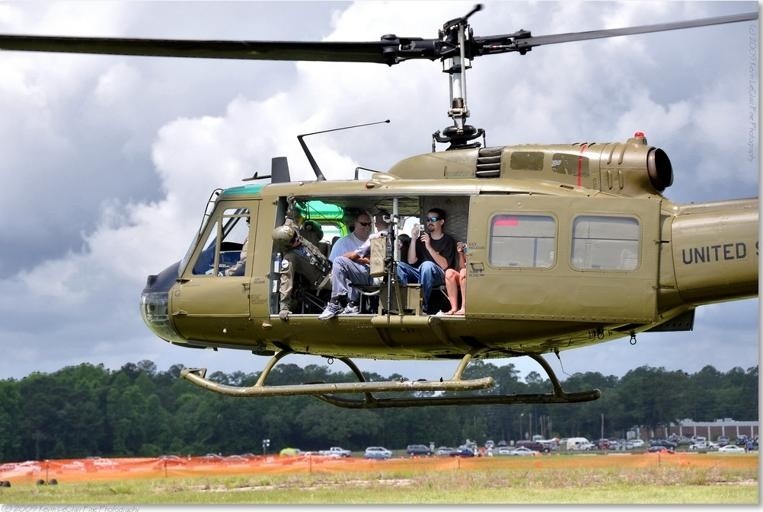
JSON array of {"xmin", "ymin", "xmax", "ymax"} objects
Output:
[
  {"xmin": 519, "ymin": 413, "xmax": 524, "ymax": 440},
  {"xmin": 262, "ymin": 438, "xmax": 271, "ymax": 460}
]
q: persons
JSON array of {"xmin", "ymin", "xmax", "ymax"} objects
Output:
[
  {"xmin": 271, "ymin": 193, "xmax": 467, "ymax": 321},
  {"xmin": 205, "ymin": 213, "xmax": 250, "ymax": 277}
]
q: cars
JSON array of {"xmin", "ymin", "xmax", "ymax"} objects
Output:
[
  {"xmin": 0, "ymin": 451, "xmax": 263, "ymax": 476},
  {"xmin": 278, "ymin": 446, "xmax": 352, "ymax": 460}
]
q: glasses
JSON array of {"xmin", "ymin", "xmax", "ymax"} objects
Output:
[
  {"xmin": 289, "ymin": 231, "xmax": 297, "ymax": 244},
  {"xmin": 356, "ymin": 219, "xmax": 372, "ymax": 227},
  {"xmin": 425, "ymin": 216, "xmax": 443, "ymax": 223}
]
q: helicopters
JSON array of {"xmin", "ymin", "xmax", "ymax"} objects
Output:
[{"xmin": 0, "ymin": 3, "xmax": 758, "ymax": 409}]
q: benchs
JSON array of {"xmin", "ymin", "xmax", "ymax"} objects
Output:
[{"xmin": 347, "ymin": 281, "xmax": 386, "ymax": 314}]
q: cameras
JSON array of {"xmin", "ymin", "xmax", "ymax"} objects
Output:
[
  {"xmin": 415, "ymin": 224, "xmax": 425, "ymax": 231},
  {"xmin": 463, "ymin": 243, "xmax": 467, "ymax": 248}
]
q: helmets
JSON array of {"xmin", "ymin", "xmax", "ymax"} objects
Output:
[
  {"xmin": 272, "ymin": 226, "xmax": 295, "ymax": 253},
  {"xmin": 300, "ymin": 220, "xmax": 323, "ymax": 240}
]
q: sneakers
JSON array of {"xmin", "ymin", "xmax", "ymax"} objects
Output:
[
  {"xmin": 340, "ymin": 302, "xmax": 360, "ymax": 314},
  {"xmin": 318, "ymin": 301, "xmax": 344, "ymax": 321},
  {"xmin": 444, "ymin": 308, "xmax": 465, "ymax": 315}
]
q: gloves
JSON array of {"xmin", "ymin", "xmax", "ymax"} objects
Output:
[{"xmin": 279, "ymin": 310, "xmax": 293, "ymax": 321}]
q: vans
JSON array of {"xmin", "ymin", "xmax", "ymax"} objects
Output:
[
  {"xmin": 406, "ymin": 444, "xmax": 431, "ymax": 456},
  {"xmin": 363, "ymin": 446, "xmax": 392, "ymax": 459}
]
